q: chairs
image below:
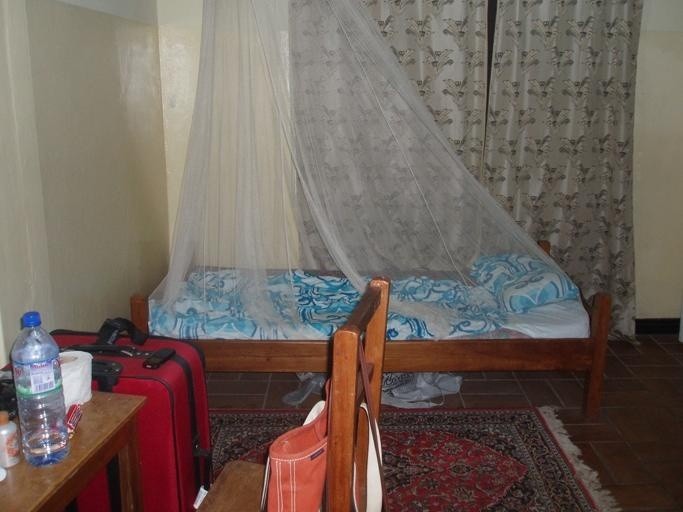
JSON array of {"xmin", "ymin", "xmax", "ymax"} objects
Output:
[{"xmin": 195, "ymin": 276, "xmax": 392, "ymax": 512}]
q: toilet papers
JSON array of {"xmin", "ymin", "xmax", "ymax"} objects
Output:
[{"xmin": 57, "ymin": 350, "xmax": 93, "ymax": 412}]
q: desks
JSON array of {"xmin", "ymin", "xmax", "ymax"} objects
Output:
[{"xmin": 0, "ymin": 388, "xmax": 151, "ymax": 510}]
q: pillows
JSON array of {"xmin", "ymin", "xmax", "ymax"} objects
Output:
[{"xmin": 471, "ymin": 249, "xmax": 579, "ymax": 314}]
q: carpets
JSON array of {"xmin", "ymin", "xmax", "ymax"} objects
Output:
[{"xmin": 205, "ymin": 399, "xmax": 622, "ymax": 512}]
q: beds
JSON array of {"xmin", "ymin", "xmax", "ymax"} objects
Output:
[{"xmin": 125, "ymin": 261, "xmax": 611, "ymax": 421}]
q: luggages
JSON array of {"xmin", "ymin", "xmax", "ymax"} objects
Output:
[{"xmin": 0, "ymin": 318, "xmax": 212, "ymax": 512}]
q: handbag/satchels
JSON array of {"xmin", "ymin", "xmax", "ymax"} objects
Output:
[{"xmin": 267, "ymin": 378, "xmax": 328, "ymax": 512}]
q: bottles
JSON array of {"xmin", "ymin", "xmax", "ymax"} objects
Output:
[{"xmin": 9, "ymin": 311, "xmax": 69, "ymax": 467}]
